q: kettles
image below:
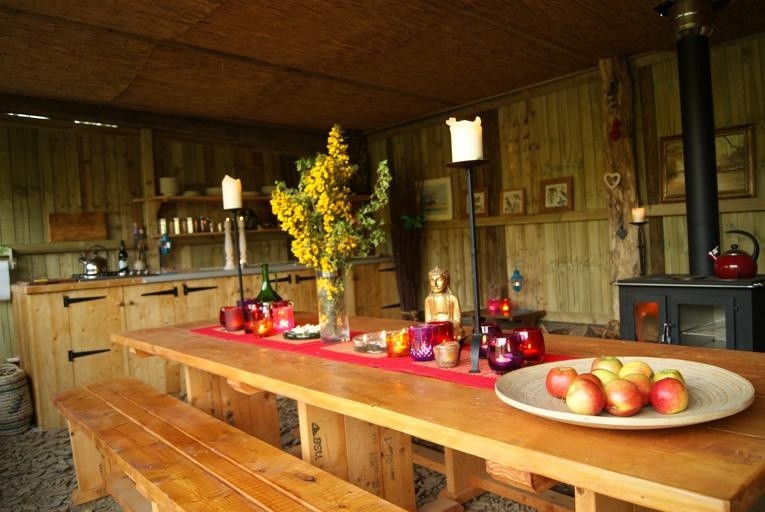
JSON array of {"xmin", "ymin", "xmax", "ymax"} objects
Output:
[
  {"xmin": 77, "ymin": 243, "xmax": 109, "ymax": 275},
  {"xmin": 706, "ymin": 229, "xmax": 760, "ymax": 279}
]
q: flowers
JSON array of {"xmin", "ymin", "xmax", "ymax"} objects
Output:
[{"xmin": 268, "ymin": 122, "xmax": 395, "ymax": 332}]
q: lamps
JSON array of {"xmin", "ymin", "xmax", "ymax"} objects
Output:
[{"xmin": 511, "ymin": 259, "xmax": 531, "ymax": 313}]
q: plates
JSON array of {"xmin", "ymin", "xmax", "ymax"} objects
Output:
[
  {"xmin": 352, "ymin": 331, "xmax": 393, "ymax": 354},
  {"xmin": 158, "ymin": 176, "xmax": 276, "ymax": 196},
  {"xmin": 495, "ymin": 355, "xmax": 755, "ymax": 430},
  {"xmin": 284, "ymin": 328, "xmax": 320, "ymax": 340}
]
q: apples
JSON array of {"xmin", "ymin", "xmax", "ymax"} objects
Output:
[{"xmin": 547, "ymin": 355, "xmax": 690, "ymax": 415}]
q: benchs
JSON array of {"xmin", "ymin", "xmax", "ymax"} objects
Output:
[{"xmin": 52, "ymin": 376, "xmax": 408, "ymax": 512}]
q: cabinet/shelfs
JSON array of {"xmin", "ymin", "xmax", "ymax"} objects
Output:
[{"xmin": 132, "ymin": 195, "xmax": 283, "ymax": 269}]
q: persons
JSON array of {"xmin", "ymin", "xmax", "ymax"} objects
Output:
[{"xmin": 421, "ymin": 263, "xmax": 464, "ymax": 341}]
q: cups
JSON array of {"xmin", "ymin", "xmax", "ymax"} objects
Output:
[
  {"xmin": 433, "ymin": 341, "xmax": 460, "ymax": 367},
  {"xmin": 221, "ymin": 296, "xmax": 294, "ymax": 338},
  {"xmin": 485, "ymin": 297, "xmax": 513, "ymax": 317},
  {"xmin": 512, "ymin": 327, "xmax": 545, "ymax": 366},
  {"xmin": 485, "ymin": 333, "xmax": 524, "ymax": 375},
  {"xmin": 386, "ymin": 322, "xmax": 455, "ymax": 362},
  {"xmin": 473, "ymin": 322, "xmax": 502, "ymax": 359}
]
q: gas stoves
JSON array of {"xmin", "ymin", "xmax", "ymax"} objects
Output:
[{"xmin": 71, "ymin": 270, "xmax": 158, "ymax": 282}]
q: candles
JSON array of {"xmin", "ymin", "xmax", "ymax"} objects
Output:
[
  {"xmin": 446, "ymin": 115, "xmax": 484, "ymax": 163},
  {"xmin": 224, "ymin": 217, "xmax": 232, "ymax": 229},
  {"xmin": 221, "ymin": 174, "xmax": 244, "ymax": 211},
  {"xmin": 237, "ymin": 217, "xmax": 246, "ymax": 230},
  {"xmin": 632, "ymin": 207, "xmax": 646, "ymax": 223}
]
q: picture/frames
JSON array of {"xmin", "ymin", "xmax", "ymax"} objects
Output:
[
  {"xmin": 500, "ymin": 188, "xmax": 526, "ymax": 216},
  {"xmin": 415, "ymin": 177, "xmax": 453, "ymax": 221},
  {"xmin": 539, "ymin": 176, "xmax": 574, "ymax": 213},
  {"xmin": 462, "ymin": 187, "xmax": 489, "ymax": 217},
  {"xmin": 659, "ymin": 123, "xmax": 758, "ymax": 204}
]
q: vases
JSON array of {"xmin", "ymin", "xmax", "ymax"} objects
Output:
[{"xmin": 314, "ymin": 268, "xmax": 350, "ymax": 345}]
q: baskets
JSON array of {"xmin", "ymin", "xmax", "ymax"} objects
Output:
[{"xmin": 0, "ymin": 361, "xmax": 35, "ymax": 437}]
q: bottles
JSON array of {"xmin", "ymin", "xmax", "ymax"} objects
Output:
[
  {"xmin": 118, "ymin": 239, "xmax": 128, "ymax": 272},
  {"xmin": 241, "ymin": 208, "xmax": 257, "ymax": 230},
  {"xmin": 132, "ymin": 221, "xmax": 144, "ymax": 240},
  {"xmin": 256, "ymin": 264, "xmax": 283, "ymax": 308},
  {"xmin": 158, "ymin": 215, "xmax": 225, "ymax": 234}
]
